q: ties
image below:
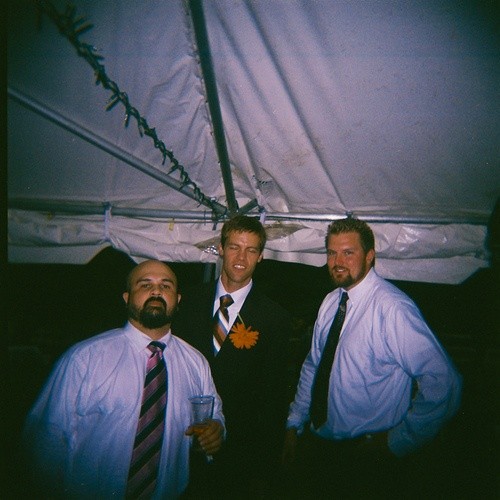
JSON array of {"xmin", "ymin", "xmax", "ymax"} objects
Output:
[
  {"xmin": 127, "ymin": 341, "xmax": 168, "ymax": 500},
  {"xmin": 211, "ymin": 295, "xmax": 235, "ymax": 356},
  {"xmin": 309, "ymin": 292, "xmax": 350, "ymax": 430}
]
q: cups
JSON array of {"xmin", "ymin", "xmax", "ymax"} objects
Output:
[{"xmin": 189, "ymin": 396, "xmax": 215, "ymax": 448}]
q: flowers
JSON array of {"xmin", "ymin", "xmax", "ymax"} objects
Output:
[{"xmin": 228, "ymin": 313, "xmax": 260, "ymax": 350}]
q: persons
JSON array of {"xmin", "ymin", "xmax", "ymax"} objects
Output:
[
  {"xmin": 283, "ymin": 219, "xmax": 462, "ymax": 500},
  {"xmin": 18, "ymin": 259, "xmax": 227, "ymax": 500},
  {"xmin": 170, "ymin": 212, "xmax": 293, "ymax": 500}
]
